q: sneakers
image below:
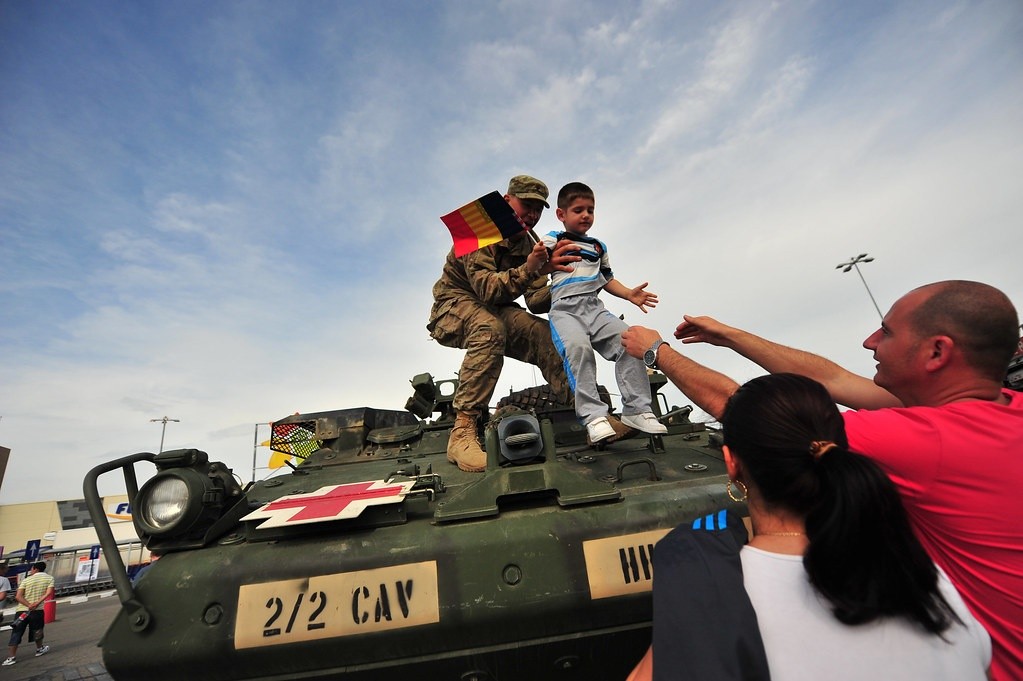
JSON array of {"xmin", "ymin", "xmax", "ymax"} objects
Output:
[
  {"xmin": 587, "ymin": 417, "xmax": 616, "ymax": 443},
  {"xmin": 1, "ymin": 657, "xmax": 16, "ymax": 666},
  {"xmin": 36, "ymin": 644, "xmax": 49, "ymax": 656},
  {"xmin": 620, "ymin": 413, "xmax": 668, "ymax": 433}
]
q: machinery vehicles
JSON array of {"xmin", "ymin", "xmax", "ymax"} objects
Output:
[{"xmin": 81, "ymin": 373, "xmax": 756, "ymax": 681}]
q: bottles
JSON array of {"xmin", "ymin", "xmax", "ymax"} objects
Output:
[{"xmin": 10, "ymin": 609, "xmax": 33, "ymax": 630}]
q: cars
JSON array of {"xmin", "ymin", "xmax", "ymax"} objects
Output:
[{"xmin": 1003, "ymin": 355, "xmax": 1023, "ymax": 392}]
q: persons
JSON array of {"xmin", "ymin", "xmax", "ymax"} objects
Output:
[
  {"xmin": 621, "ymin": 280, "xmax": 1023, "ymax": 681},
  {"xmin": 625, "ymin": 372, "xmax": 994, "ymax": 681},
  {"xmin": 527, "ymin": 182, "xmax": 669, "ymax": 443},
  {"xmin": 2, "ymin": 562, "xmax": 55, "ymax": 667},
  {"xmin": 0, "ymin": 563, "xmax": 11, "ymax": 602},
  {"xmin": 426, "ymin": 175, "xmax": 632, "ymax": 472}
]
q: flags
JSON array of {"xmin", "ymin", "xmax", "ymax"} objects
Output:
[{"xmin": 439, "ymin": 189, "xmax": 524, "ymax": 259}]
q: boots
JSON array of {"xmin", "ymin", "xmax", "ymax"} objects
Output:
[
  {"xmin": 586, "ymin": 411, "xmax": 631, "ymax": 446},
  {"xmin": 446, "ymin": 410, "xmax": 487, "ymax": 472}
]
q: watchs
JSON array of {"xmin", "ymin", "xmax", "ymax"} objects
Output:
[{"xmin": 643, "ymin": 341, "xmax": 669, "ymax": 367}]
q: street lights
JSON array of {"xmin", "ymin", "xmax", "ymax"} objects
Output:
[
  {"xmin": 150, "ymin": 416, "xmax": 179, "ymax": 454},
  {"xmin": 836, "ymin": 254, "xmax": 885, "ymax": 322}
]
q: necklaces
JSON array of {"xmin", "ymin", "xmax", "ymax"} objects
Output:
[{"xmin": 753, "ymin": 531, "xmax": 807, "ymax": 539}]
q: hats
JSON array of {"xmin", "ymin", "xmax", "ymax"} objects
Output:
[{"xmin": 508, "ymin": 175, "xmax": 550, "ymax": 209}]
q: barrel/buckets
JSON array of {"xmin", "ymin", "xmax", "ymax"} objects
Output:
[
  {"xmin": 44, "ymin": 600, "xmax": 56, "ymax": 623},
  {"xmin": 45, "ymin": 589, "xmax": 54, "ymax": 600}
]
